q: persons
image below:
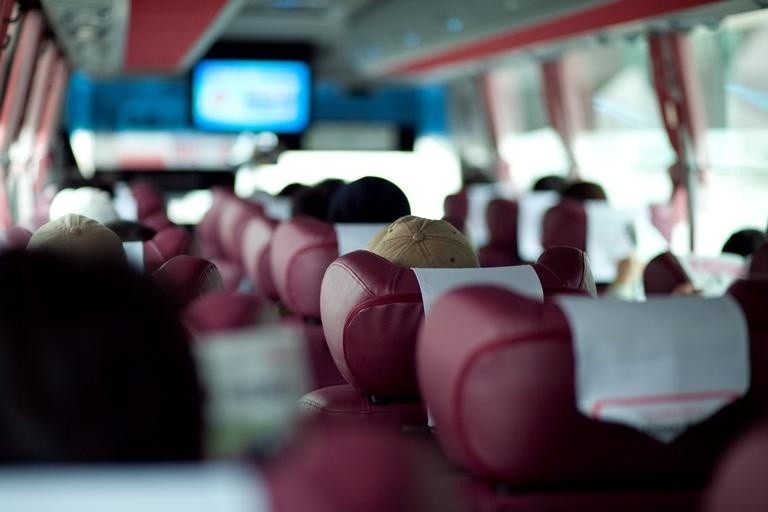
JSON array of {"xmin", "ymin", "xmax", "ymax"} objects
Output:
[
  {"xmin": 49, "ymin": 186, "xmax": 119, "ymax": 224},
  {"xmin": 0, "ymin": 250, "xmax": 207, "ymax": 462},
  {"xmin": 327, "ymin": 177, "xmax": 410, "ymax": 223},
  {"xmin": 300, "ymin": 179, "xmax": 346, "ymax": 222},
  {"xmin": 562, "ymin": 183, "xmax": 606, "ymax": 199},
  {"xmin": 279, "ymin": 184, "xmax": 302, "ymax": 195},
  {"xmin": 722, "ymin": 230, "xmax": 763, "ymax": 256},
  {"xmin": 105, "ymin": 221, "xmax": 156, "ymax": 241},
  {"xmin": 534, "ymin": 177, "xmax": 565, "ymax": 190},
  {"xmin": 598, "ymin": 258, "xmax": 641, "ymax": 299}
]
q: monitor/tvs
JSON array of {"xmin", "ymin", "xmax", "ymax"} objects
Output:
[{"xmin": 185, "ymin": 54, "xmax": 314, "ymax": 136}]
